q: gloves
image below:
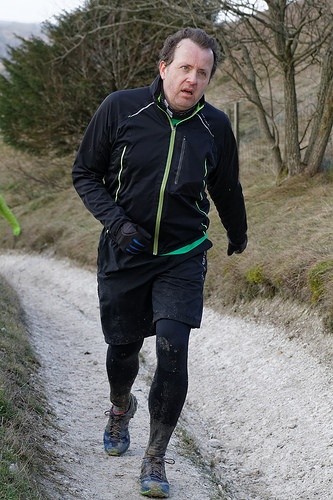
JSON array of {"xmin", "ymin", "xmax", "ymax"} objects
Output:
[
  {"xmin": 227, "ymin": 232, "xmax": 247, "ymax": 256},
  {"xmin": 114, "ymin": 222, "xmax": 152, "ymax": 256}
]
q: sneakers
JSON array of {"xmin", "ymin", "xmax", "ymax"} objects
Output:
[
  {"xmin": 104, "ymin": 393, "xmax": 138, "ymax": 455},
  {"xmin": 139, "ymin": 453, "xmax": 175, "ymax": 498}
]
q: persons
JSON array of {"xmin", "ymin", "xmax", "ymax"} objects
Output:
[{"xmin": 71, "ymin": 27, "xmax": 249, "ymax": 499}]
